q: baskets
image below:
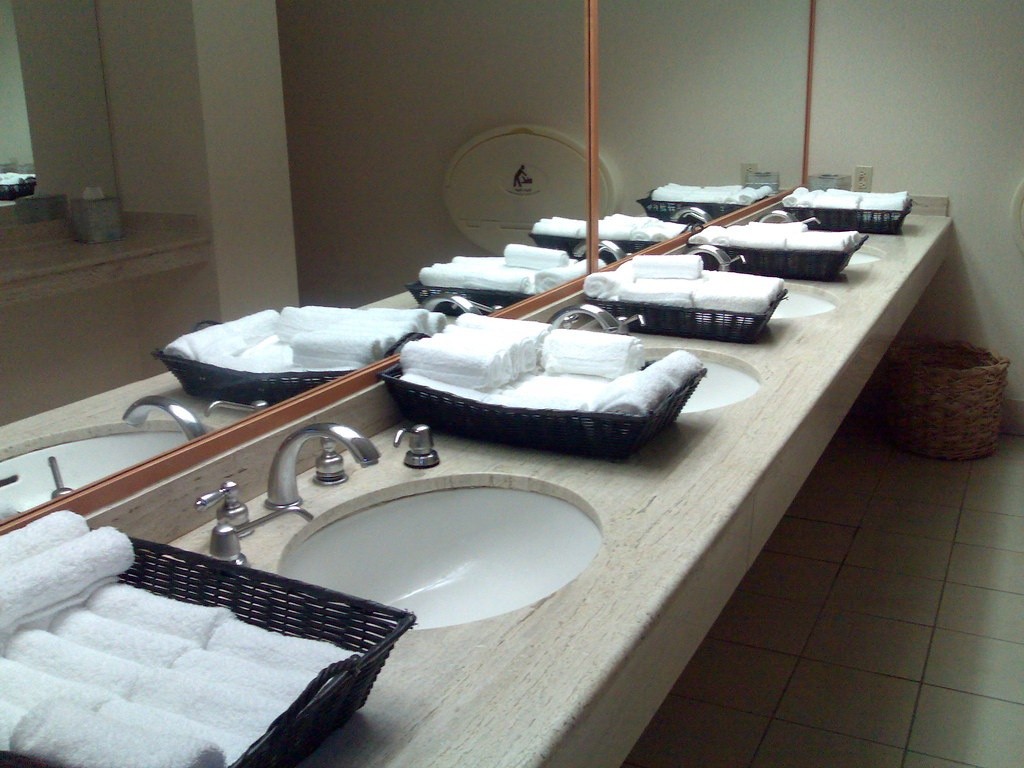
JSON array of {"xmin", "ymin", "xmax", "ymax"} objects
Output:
[
  {"xmin": 529, "ymin": 233, "xmax": 662, "ymax": 267},
  {"xmin": 0, "ymin": 526, "xmax": 418, "ymax": 768},
  {"xmin": 584, "ymin": 288, "xmax": 788, "ymax": 345},
  {"xmin": 150, "ymin": 320, "xmax": 433, "ymax": 404},
  {"xmin": 378, "ymin": 360, "xmax": 708, "ymax": 463},
  {"xmin": 635, "ymin": 187, "xmax": 772, "ymax": 228},
  {"xmin": 882, "ymin": 333, "xmax": 1009, "ymax": 461},
  {"xmin": 782, "ymin": 199, "xmax": 914, "ymax": 234},
  {"xmin": 405, "ymin": 280, "xmax": 535, "ymax": 317},
  {"xmin": 687, "ymin": 223, "xmax": 869, "ymax": 282}
]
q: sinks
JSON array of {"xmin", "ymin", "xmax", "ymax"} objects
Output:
[
  {"xmin": 846, "ymin": 244, "xmax": 886, "ymax": 265},
  {"xmin": 644, "ymin": 347, "xmax": 762, "ymax": 412},
  {"xmin": 63, "ymin": 421, "xmax": 215, "ymax": 498},
  {"xmin": 277, "ymin": 473, "xmax": 604, "ymax": 632},
  {"xmin": 770, "ymin": 282, "xmax": 841, "ymax": 319},
  {"xmin": 0, "ymin": 422, "xmax": 213, "ymax": 525}
]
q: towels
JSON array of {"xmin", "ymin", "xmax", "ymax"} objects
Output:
[
  {"xmin": 163, "ymin": 305, "xmax": 427, "ymax": 372},
  {"xmin": 0, "ymin": 510, "xmax": 364, "ymax": 768},
  {"xmin": 689, "ymin": 221, "xmax": 860, "ymax": 252},
  {"xmin": 419, "ymin": 244, "xmax": 605, "ymax": 295},
  {"xmin": 651, "ymin": 182, "xmax": 772, "ymax": 206},
  {"xmin": 782, "ymin": 186, "xmax": 912, "ymax": 210},
  {"xmin": 399, "ymin": 313, "xmax": 702, "ymax": 417},
  {"xmin": 583, "ymin": 255, "xmax": 785, "ymax": 313},
  {"xmin": 531, "ymin": 213, "xmax": 687, "ymax": 242}
]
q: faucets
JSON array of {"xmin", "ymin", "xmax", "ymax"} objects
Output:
[
  {"xmin": 122, "ymin": 395, "xmax": 206, "ymax": 441},
  {"xmin": 669, "ymin": 206, "xmax": 712, "ymax": 223},
  {"xmin": 418, "ymin": 294, "xmax": 482, "ymax": 317},
  {"xmin": 267, "ymin": 423, "xmax": 381, "ymax": 506},
  {"xmin": 754, "ymin": 210, "xmax": 795, "ymax": 222},
  {"xmin": 546, "ymin": 304, "xmax": 620, "ymax": 332},
  {"xmin": 682, "ymin": 245, "xmax": 730, "ymax": 267},
  {"xmin": 573, "ymin": 239, "xmax": 624, "ymax": 260}
]
